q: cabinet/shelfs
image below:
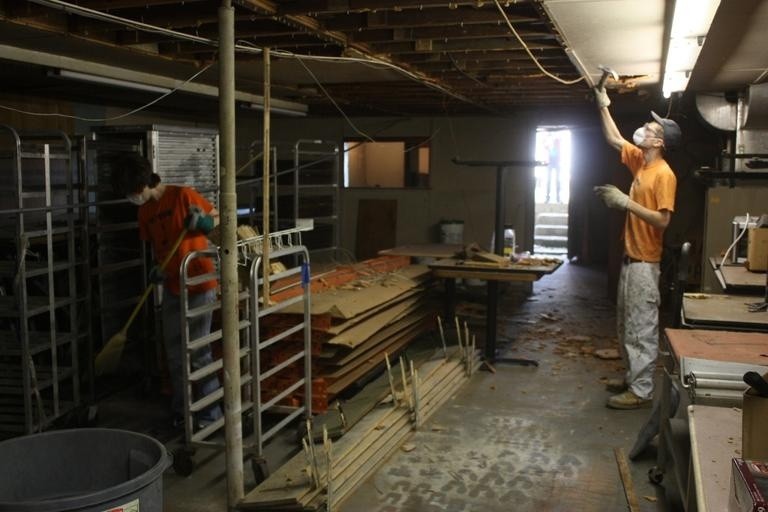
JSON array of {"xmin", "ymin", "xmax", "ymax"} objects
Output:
[
  {"xmin": 250, "ymin": 139, "xmax": 342, "ymax": 273},
  {"xmin": 0, "ymin": 120, "xmax": 155, "ymax": 436}
]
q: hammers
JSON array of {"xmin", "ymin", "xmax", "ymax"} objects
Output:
[{"xmin": 596, "ymin": 64, "xmax": 619, "ymax": 93}]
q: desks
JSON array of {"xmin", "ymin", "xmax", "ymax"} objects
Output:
[{"xmin": 432, "ymin": 267, "xmax": 545, "ymax": 366}]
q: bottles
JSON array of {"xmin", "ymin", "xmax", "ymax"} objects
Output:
[{"xmin": 503, "ymin": 225, "xmax": 515, "ymax": 259}]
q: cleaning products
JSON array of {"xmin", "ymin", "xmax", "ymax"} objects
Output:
[{"xmin": 504, "ymin": 223, "xmax": 516, "ymax": 254}]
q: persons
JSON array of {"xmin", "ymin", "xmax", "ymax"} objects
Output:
[
  {"xmin": 545, "ymin": 139, "xmax": 560, "ymax": 204},
  {"xmin": 111, "ymin": 152, "xmax": 223, "ymax": 427},
  {"xmin": 594, "ymin": 87, "xmax": 682, "ymax": 409}
]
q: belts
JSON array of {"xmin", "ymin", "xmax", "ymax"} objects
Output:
[{"xmin": 621, "ymin": 253, "xmax": 654, "ymax": 266}]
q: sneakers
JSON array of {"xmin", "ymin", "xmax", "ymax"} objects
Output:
[{"xmin": 602, "ymin": 376, "xmax": 656, "ymax": 412}]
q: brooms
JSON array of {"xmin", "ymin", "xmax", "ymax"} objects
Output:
[{"xmin": 88, "ymin": 206, "xmax": 203, "ymax": 390}]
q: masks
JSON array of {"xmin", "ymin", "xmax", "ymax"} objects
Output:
[{"xmin": 125, "ymin": 195, "xmax": 148, "ymax": 206}]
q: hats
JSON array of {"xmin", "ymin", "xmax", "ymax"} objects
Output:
[{"xmin": 649, "ymin": 109, "xmax": 683, "ymax": 153}]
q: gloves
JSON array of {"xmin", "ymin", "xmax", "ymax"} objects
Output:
[
  {"xmin": 147, "ymin": 259, "xmax": 172, "ymax": 285},
  {"xmin": 592, "ymin": 183, "xmax": 630, "ymax": 212},
  {"xmin": 182, "ymin": 211, "xmax": 214, "ymax": 236},
  {"xmin": 583, "ymin": 85, "xmax": 611, "ymax": 111}
]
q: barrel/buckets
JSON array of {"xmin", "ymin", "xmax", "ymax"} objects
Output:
[{"xmin": 439, "ymin": 219, "xmax": 465, "ymax": 246}]
